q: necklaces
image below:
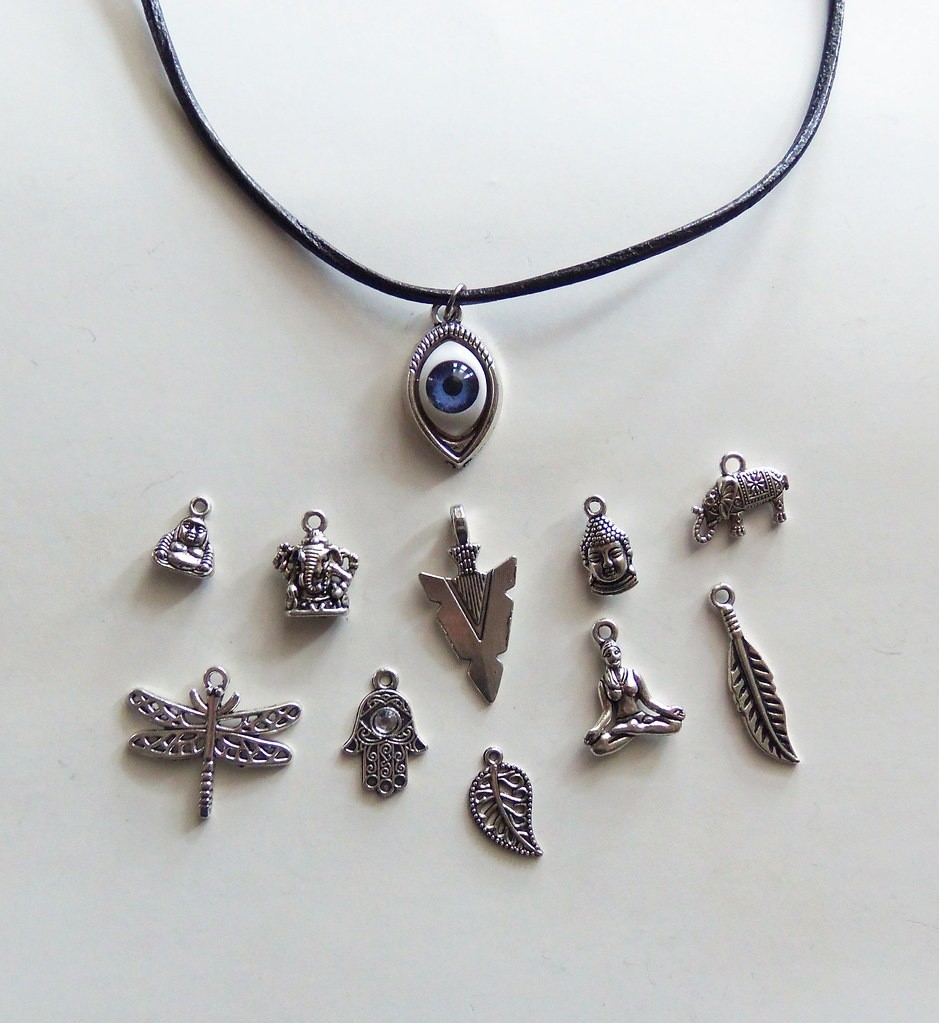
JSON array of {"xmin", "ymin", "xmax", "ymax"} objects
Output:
[{"xmin": 142, "ymin": 0, "xmax": 845, "ymax": 466}]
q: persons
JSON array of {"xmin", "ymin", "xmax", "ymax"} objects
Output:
[
  {"xmin": 584, "ymin": 641, "xmax": 685, "ymax": 756},
  {"xmin": 152, "ymin": 516, "xmax": 215, "ymax": 579},
  {"xmin": 580, "ymin": 516, "xmax": 639, "ymax": 595}
]
